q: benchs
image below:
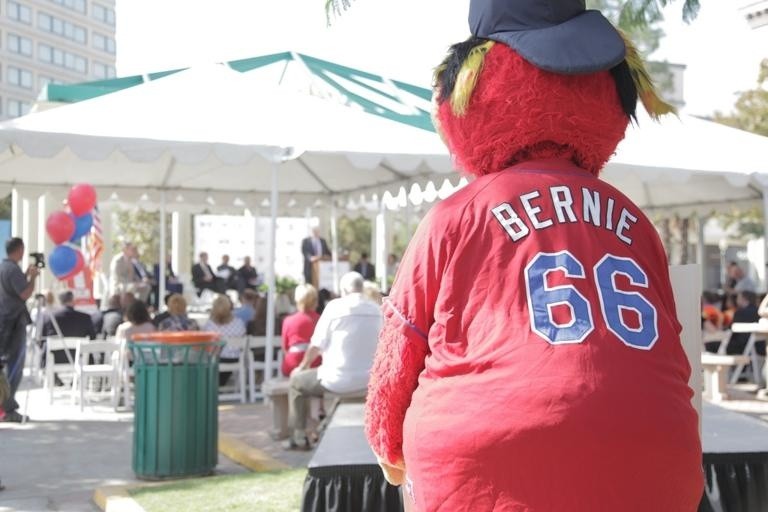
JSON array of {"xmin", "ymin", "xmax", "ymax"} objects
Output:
[
  {"xmin": 300, "ymin": 397, "xmax": 405, "ymax": 511},
  {"xmin": 703, "ymin": 397, "xmax": 768, "ymax": 511},
  {"xmin": 261, "ymin": 378, "xmax": 291, "ymax": 440},
  {"xmin": 702, "ymin": 355, "xmax": 750, "ymax": 403}
]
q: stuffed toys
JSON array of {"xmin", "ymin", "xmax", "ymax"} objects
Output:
[{"xmin": 362, "ymin": 0, "xmax": 702, "ymax": 512}]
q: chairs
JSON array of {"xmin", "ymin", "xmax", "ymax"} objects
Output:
[
  {"xmin": 99, "ymin": 272, "xmax": 111, "ymax": 308},
  {"xmin": 45, "ymin": 333, "xmax": 290, "ymax": 413}
]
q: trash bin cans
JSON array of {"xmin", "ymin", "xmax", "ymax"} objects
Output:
[{"xmin": 127, "ymin": 342, "xmax": 225, "ymax": 482}]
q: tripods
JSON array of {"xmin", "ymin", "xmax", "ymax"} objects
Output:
[{"xmin": 18, "ymin": 273, "xmax": 79, "ymax": 427}]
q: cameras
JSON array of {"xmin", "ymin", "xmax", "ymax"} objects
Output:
[{"xmin": 29, "ymin": 251, "xmax": 48, "ymax": 269}]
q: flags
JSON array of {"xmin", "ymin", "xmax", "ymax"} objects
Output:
[{"xmin": 60, "ymin": 195, "xmax": 104, "ymax": 271}]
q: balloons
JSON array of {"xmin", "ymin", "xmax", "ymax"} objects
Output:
[{"xmin": 45, "ymin": 182, "xmax": 95, "ymax": 283}]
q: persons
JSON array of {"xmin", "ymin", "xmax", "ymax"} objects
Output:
[
  {"xmin": 36, "ymin": 244, "xmax": 259, "ymax": 392},
  {"xmin": 1, "ymin": 357, "xmax": 32, "ymax": 423},
  {"xmin": 278, "ymin": 283, "xmax": 326, "ymax": 421},
  {"xmin": 316, "ymin": 251, "xmax": 398, "ymax": 318},
  {"xmin": 1, "ymin": 238, "xmax": 39, "ymax": 401},
  {"xmin": 279, "ymin": 272, "xmax": 384, "ymax": 451},
  {"xmin": 718, "ymin": 259, "xmax": 768, "ymax": 384},
  {"xmin": 300, "ymin": 226, "xmax": 332, "ymax": 282}
]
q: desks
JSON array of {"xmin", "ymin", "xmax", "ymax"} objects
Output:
[{"xmin": 703, "ymin": 323, "xmax": 768, "ymax": 388}]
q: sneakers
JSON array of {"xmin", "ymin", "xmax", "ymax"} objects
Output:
[
  {"xmin": 0, "ymin": 411, "xmax": 29, "ymax": 422},
  {"xmin": 281, "ymin": 437, "xmax": 310, "ymax": 451}
]
